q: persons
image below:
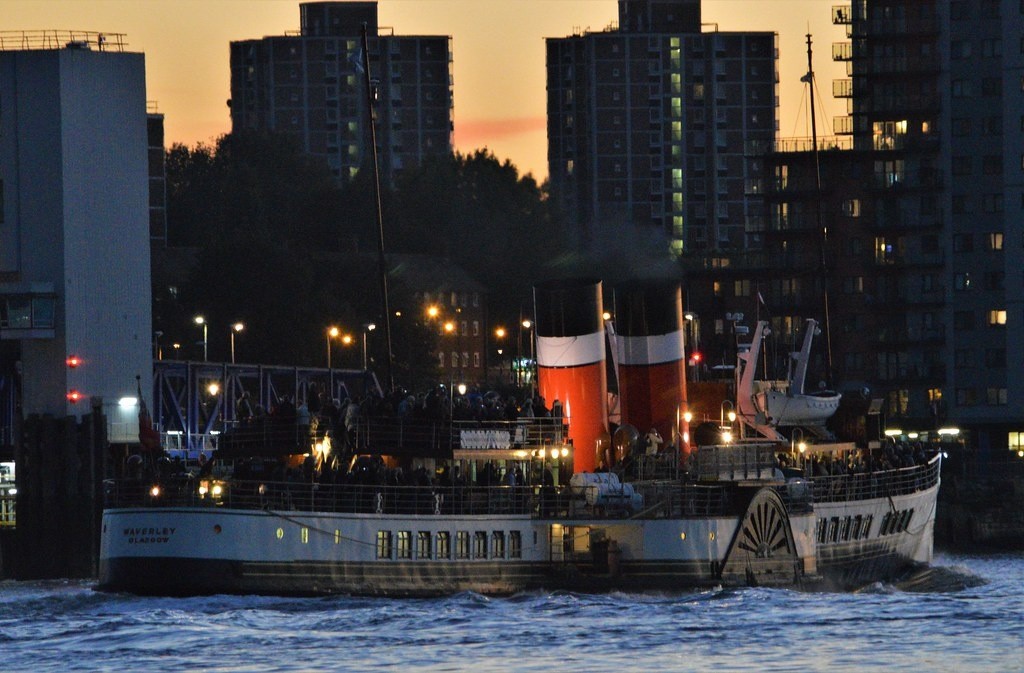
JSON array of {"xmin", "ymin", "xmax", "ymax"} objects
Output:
[
  {"xmin": 152, "ymin": 450, "xmax": 571, "ymax": 518},
  {"xmin": 235, "ymin": 382, "xmax": 555, "ymax": 444},
  {"xmin": 593, "ymin": 460, "xmax": 609, "ymax": 473},
  {"xmin": 812, "ymin": 438, "xmax": 938, "ymax": 502},
  {"xmin": 635, "ymin": 425, "xmax": 663, "ymax": 472}
]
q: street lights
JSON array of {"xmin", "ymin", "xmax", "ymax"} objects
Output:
[
  {"xmin": 231, "ymin": 320, "xmax": 244, "ymax": 364},
  {"xmin": 192, "ymin": 314, "xmax": 208, "ymax": 361},
  {"xmin": 326, "ymin": 326, "xmax": 339, "ymax": 369},
  {"xmin": 522, "ymin": 319, "xmax": 534, "ymax": 361}
]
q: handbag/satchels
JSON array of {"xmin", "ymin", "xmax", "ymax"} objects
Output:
[{"xmin": 644, "ymin": 433, "xmax": 652, "ymax": 447}]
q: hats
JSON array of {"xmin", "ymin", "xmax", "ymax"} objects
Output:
[{"xmin": 650, "ymin": 428, "xmax": 657, "ymax": 433}]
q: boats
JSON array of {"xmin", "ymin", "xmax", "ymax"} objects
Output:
[
  {"xmin": 92, "ymin": 268, "xmax": 949, "ymax": 598},
  {"xmin": 751, "ymin": 318, "xmax": 843, "ymax": 428}
]
